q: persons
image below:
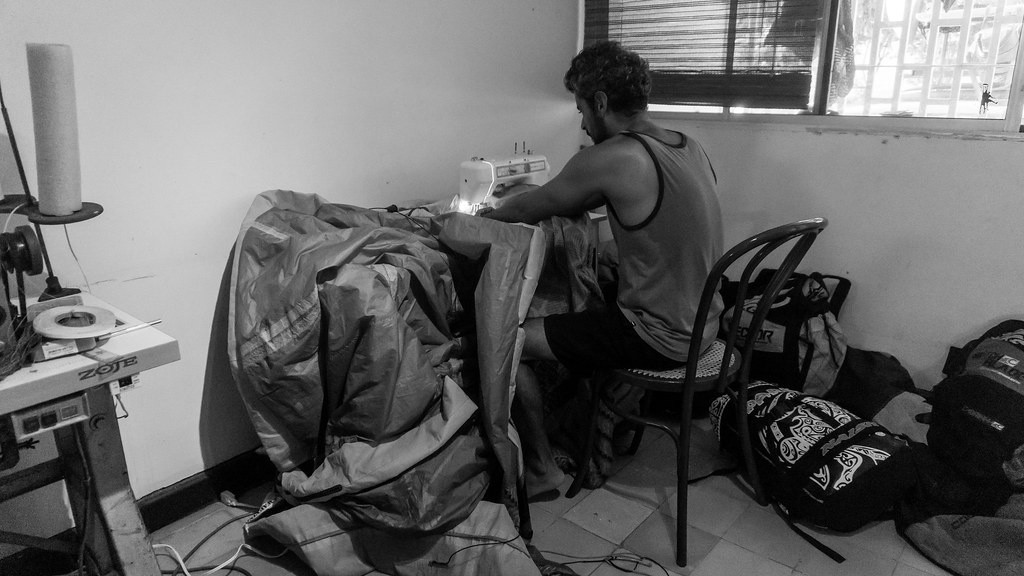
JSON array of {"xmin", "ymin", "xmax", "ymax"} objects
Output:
[{"xmin": 473, "ymin": 39, "xmax": 725, "ymax": 500}]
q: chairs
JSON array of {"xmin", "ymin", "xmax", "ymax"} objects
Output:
[{"xmin": 565, "ymin": 216, "xmax": 829, "ymax": 568}]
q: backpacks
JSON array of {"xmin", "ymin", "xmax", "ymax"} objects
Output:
[
  {"xmin": 709, "ymin": 380, "xmax": 914, "ymax": 534},
  {"xmin": 926, "ymin": 320, "xmax": 1024, "ymax": 487}
]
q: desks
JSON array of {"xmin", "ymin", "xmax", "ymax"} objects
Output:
[{"xmin": 0, "ymin": 290, "xmax": 181, "ymax": 576}]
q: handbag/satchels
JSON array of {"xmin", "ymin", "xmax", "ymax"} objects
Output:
[{"xmin": 717, "ymin": 269, "xmax": 851, "ymax": 398}]
